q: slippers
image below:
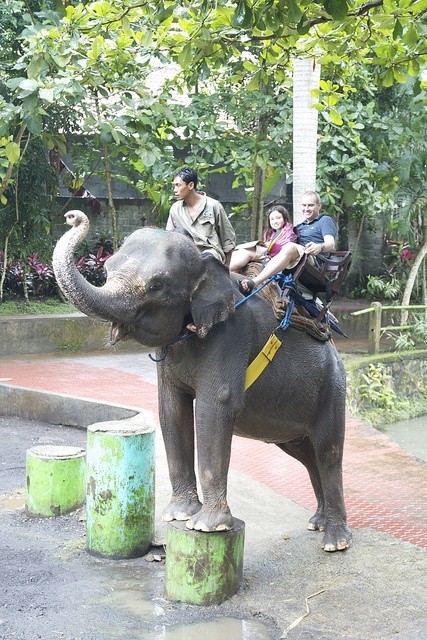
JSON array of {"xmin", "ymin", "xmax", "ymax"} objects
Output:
[{"xmin": 236, "ymin": 278, "xmax": 254, "ymax": 296}]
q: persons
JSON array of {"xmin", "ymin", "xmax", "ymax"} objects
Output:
[
  {"xmin": 163, "ymin": 166, "xmax": 238, "ymax": 336},
  {"xmin": 240, "ymin": 189, "xmax": 338, "ymax": 290},
  {"xmin": 231, "ymin": 204, "xmax": 294, "ymax": 268}
]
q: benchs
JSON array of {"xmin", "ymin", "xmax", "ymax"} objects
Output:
[{"xmin": 282, "ymin": 249, "xmax": 352, "ymax": 325}]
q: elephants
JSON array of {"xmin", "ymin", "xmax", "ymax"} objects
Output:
[{"xmin": 51, "ymin": 207, "xmax": 355, "ymax": 554}]
